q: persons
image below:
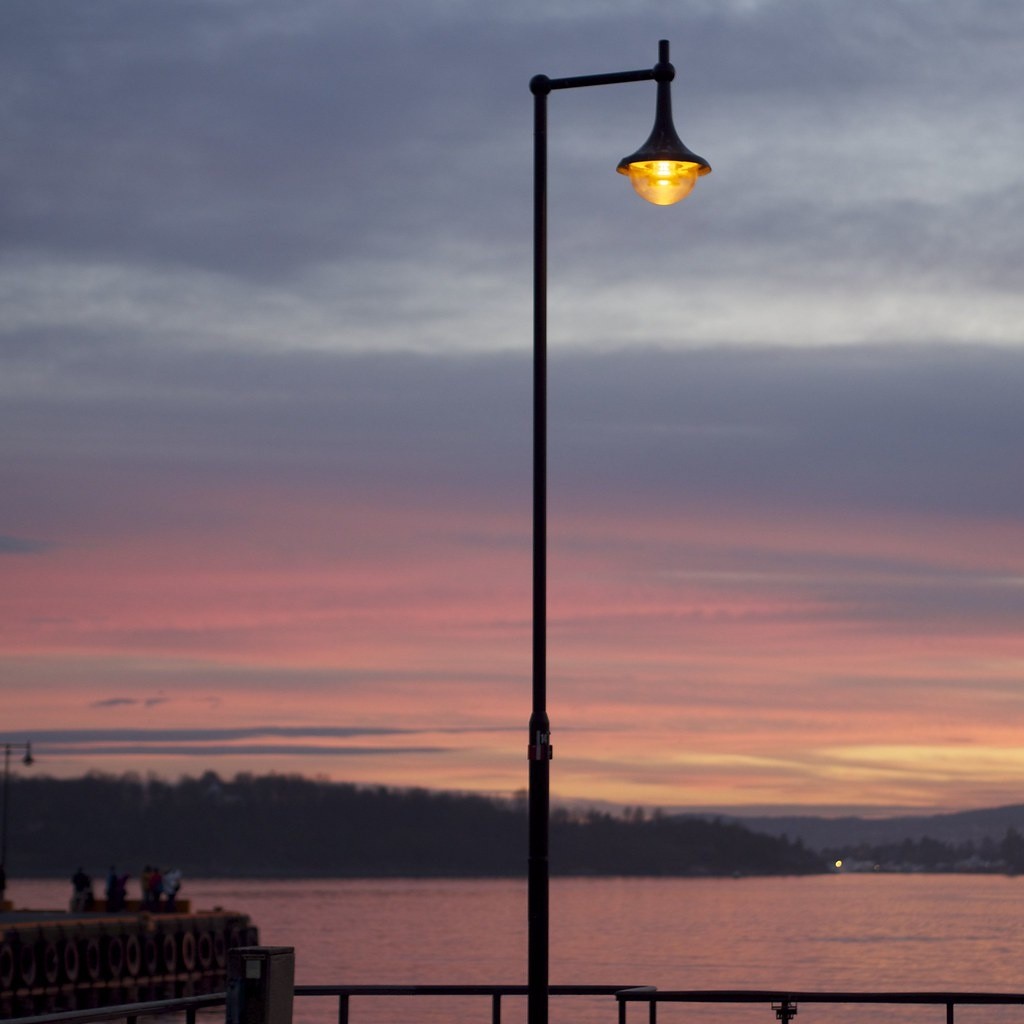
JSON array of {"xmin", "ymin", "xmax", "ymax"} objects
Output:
[
  {"xmin": 106, "ymin": 867, "xmax": 130, "ymax": 900},
  {"xmin": 71, "ymin": 868, "xmax": 93, "ymax": 899},
  {"xmin": 141, "ymin": 864, "xmax": 181, "ymax": 900}
]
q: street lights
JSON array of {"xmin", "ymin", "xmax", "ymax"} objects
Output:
[{"xmin": 528, "ymin": 37, "xmax": 713, "ymax": 1024}]
